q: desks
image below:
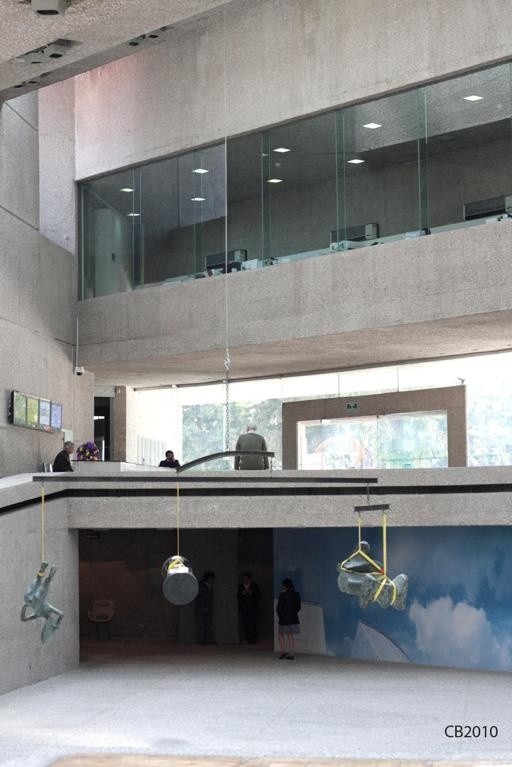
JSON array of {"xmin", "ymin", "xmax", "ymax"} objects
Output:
[{"xmin": 70, "ymin": 457, "xmax": 178, "ymax": 473}]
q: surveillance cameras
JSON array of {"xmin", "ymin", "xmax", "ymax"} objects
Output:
[{"xmin": 74, "ymin": 367, "xmax": 85, "ymax": 376}]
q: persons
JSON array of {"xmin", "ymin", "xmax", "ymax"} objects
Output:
[
  {"xmin": 52, "ymin": 439, "xmax": 74, "ymax": 471},
  {"xmin": 195, "ymin": 570, "xmax": 217, "ymax": 643},
  {"xmin": 236, "ymin": 573, "xmax": 259, "ymax": 641},
  {"xmin": 275, "ymin": 578, "xmax": 301, "ymax": 658},
  {"xmin": 159, "ymin": 449, "xmax": 180, "ymax": 468},
  {"xmin": 235, "ymin": 423, "xmax": 270, "ymax": 469}
]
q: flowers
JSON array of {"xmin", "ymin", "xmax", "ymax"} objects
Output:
[{"xmin": 75, "ymin": 441, "xmax": 102, "ymax": 462}]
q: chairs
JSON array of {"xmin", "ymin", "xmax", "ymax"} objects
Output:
[
  {"xmin": 42, "ymin": 461, "xmax": 52, "ymax": 473},
  {"xmin": 88, "ymin": 598, "xmax": 115, "ymax": 639}
]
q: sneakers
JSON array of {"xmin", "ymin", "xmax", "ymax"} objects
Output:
[{"xmin": 279, "ymin": 652, "xmax": 295, "ymax": 660}]
